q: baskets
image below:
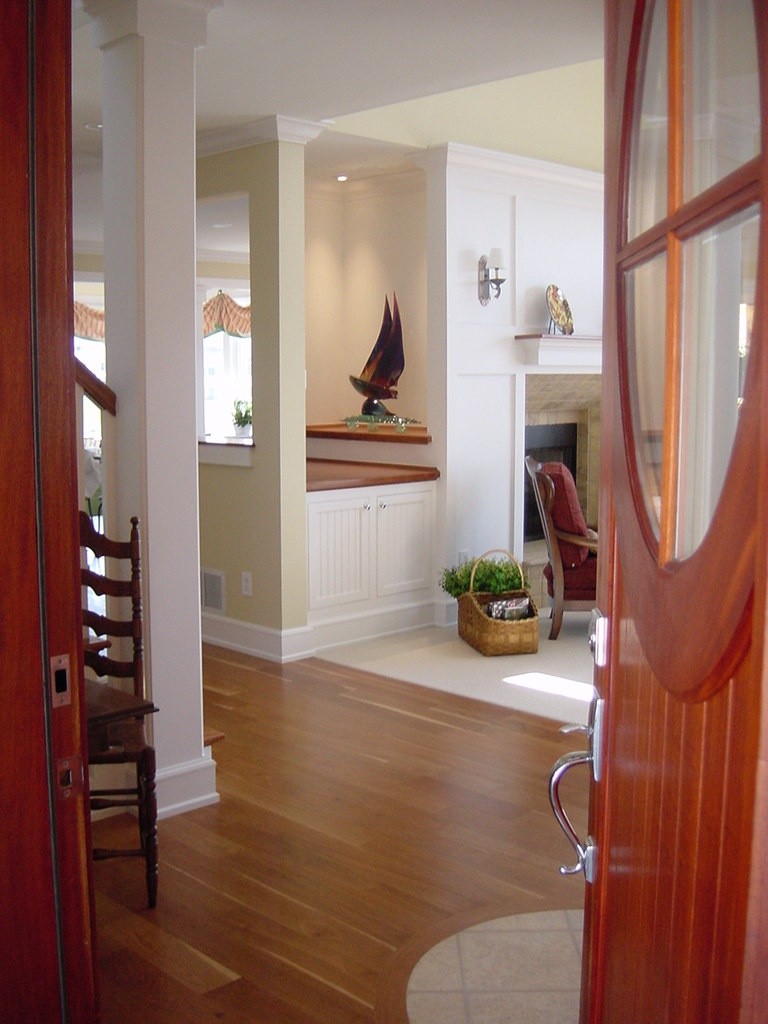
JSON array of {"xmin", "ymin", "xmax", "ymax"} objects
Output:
[{"xmin": 458, "ymin": 549, "xmax": 538, "ymax": 656}]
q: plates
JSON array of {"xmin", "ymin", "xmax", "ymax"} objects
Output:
[{"xmin": 546, "ymin": 284, "xmax": 573, "ymax": 334}]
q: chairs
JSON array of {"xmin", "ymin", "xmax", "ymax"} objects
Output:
[
  {"xmin": 79, "ymin": 511, "xmax": 161, "ymax": 909},
  {"xmin": 524, "ymin": 453, "xmax": 600, "ymax": 642}
]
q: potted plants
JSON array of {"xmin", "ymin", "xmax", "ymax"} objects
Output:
[{"xmin": 231, "ymin": 400, "xmax": 253, "ymax": 440}]
